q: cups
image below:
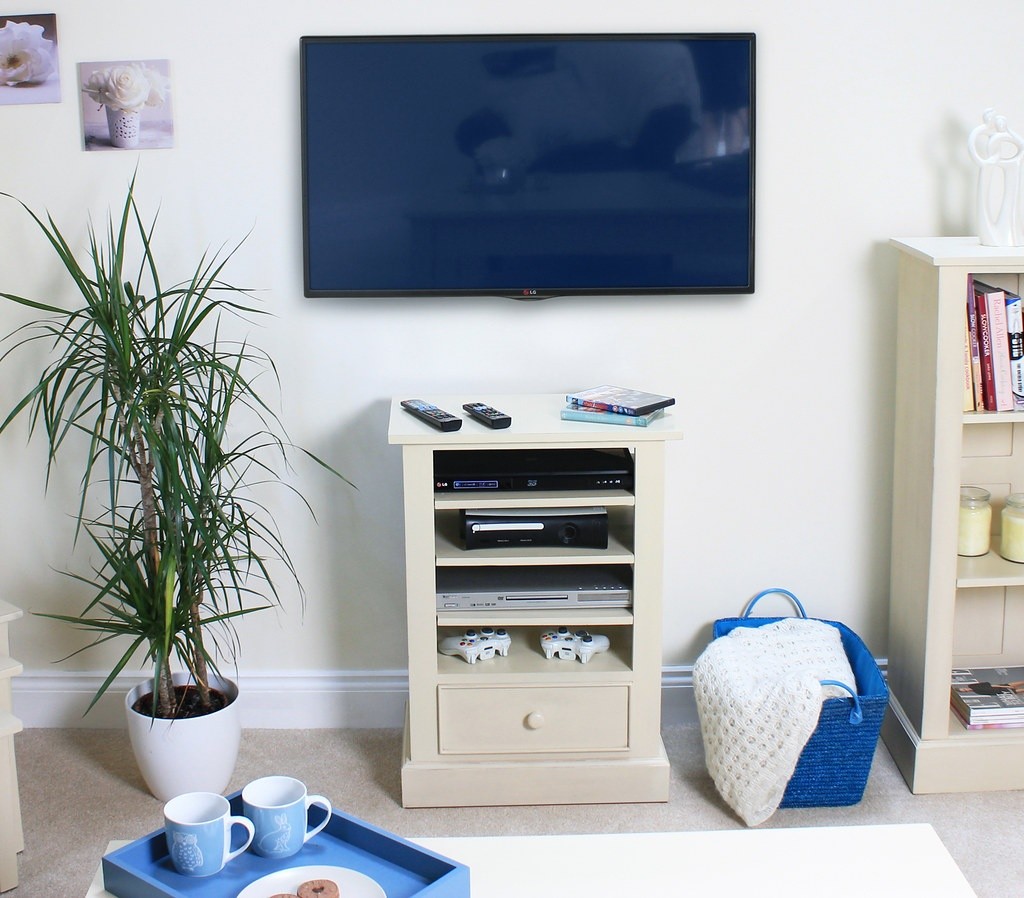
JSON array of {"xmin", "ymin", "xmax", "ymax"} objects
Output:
[
  {"xmin": 242, "ymin": 776, "xmax": 332, "ymax": 859},
  {"xmin": 162, "ymin": 792, "xmax": 255, "ymax": 878}
]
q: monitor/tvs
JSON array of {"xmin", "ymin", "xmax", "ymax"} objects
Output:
[{"xmin": 300, "ymin": 31, "xmax": 757, "ymax": 303}]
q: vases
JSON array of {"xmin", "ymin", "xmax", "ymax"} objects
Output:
[{"xmin": 104, "ymin": 104, "xmax": 141, "ymax": 148}]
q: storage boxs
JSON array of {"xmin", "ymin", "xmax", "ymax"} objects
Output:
[{"xmin": 713, "ymin": 588, "xmax": 889, "ymax": 810}]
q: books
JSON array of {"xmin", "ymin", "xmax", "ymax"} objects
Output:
[
  {"xmin": 994, "ymin": 286, "xmax": 1024, "ymax": 411},
  {"xmin": 966, "ymin": 272, "xmax": 996, "ymax": 413},
  {"xmin": 561, "ymin": 398, "xmax": 666, "ymax": 427},
  {"xmin": 565, "ymin": 385, "xmax": 676, "ymax": 413},
  {"xmin": 951, "ymin": 664, "xmax": 1023, "ymax": 730},
  {"xmin": 973, "ymin": 278, "xmax": 1014, "ymax": 411}
]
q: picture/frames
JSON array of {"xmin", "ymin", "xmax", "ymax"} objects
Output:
[
  {"xmin": 79, "ymin": 60, "xmax": 174, "ymax": 151},
  {"xmin": 0, "ymin": 13, "xmax": 60, "ymax": 105}
]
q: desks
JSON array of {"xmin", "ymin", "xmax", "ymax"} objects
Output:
[{"xmin": 83, "ymin": 821, "xmax": 987, "ymax": 898}]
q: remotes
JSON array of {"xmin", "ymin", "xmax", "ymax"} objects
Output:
[
  {"xmin": 463, "ymin": 402, "xmax": 512, "ymax": 429},
  {"xmin": 401, "ymin": 399, "xmax": 462, "ymax": 432}
]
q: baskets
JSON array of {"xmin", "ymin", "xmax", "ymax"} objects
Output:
[{"xmin": 710, "ymin": 590, "xmax": 889, "ymax": 809}]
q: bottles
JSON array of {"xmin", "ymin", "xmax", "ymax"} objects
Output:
[
  {"xmin": 999, "ymin": 493, "xmax": 1024, "ymax": 563},
  {"xmin": 958, "ymin": 485, "xmax": 992, "ymax": 556}
]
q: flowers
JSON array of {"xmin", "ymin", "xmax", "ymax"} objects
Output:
[
  {"xmin": 1, "ymin": 20, "xmax": 54, "ymax": 88},
  {"xmin": 82, "ymin": 62, "xmax": 168, "ymax": 113}
]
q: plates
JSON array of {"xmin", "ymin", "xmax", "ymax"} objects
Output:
[{"xmin": 236, "ymin": 866, "xmax": 387, "ymax": 898}]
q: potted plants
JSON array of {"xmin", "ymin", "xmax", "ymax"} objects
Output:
[{"xmin": 0, "ymin": 152, "xmax": 360, "ymax": 804}]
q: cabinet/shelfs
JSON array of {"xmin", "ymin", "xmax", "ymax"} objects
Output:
[
  {"xmin": 385, "ymin": 394, "xmax": 669, "ymax": 808},
  {"xmin": 879, "ymin": 232, "xmax": 1024, "ymax": 794}
]
